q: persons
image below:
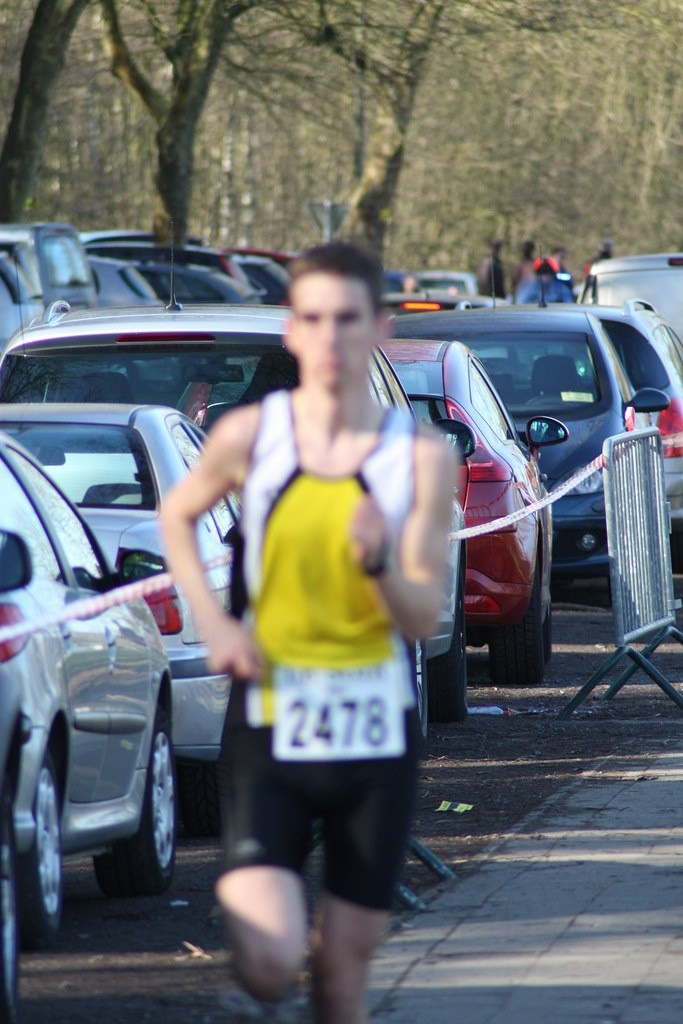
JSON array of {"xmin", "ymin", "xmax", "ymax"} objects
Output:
[
  {"xmin": 475, "ymin": 239, "xmax": 506, "ymax": 298},
  {"xmin": 550, "ymin": 247, "xmax": 573, "ymax": 288},
  {"xmin": 158, "ymin": 240, "xmax": 456, "ymax": 1024},
  {"xmin": 594, "ymin": 239, "xmax": 612, "ymax": 258},
  {"xmin": 510, "ymin": 241, "xmax": 533, "ymax": 298},
  {"xmin": 519, "ymin": 257, "xmax": 573, "ymax": 304}
]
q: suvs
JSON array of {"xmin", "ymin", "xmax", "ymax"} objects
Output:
[
  {"xmin": 498, "ymin": 298, "xmax": 683, "ymax": 545},
  {"xmin": 0, "ymin": 299, "xmax": 471, "ymax": 748},
  {"xmin": 576, "ymin": 252, "xmax": 683, "ymax": 342}
]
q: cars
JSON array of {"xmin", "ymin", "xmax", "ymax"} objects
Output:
[
  {"xmin": 0, "ymin": 218, "xmax": 507, "ymax": 348},
  {"xmin": 0, "ymin": 439, "xmax": 194, "ymax": 955},
  {"xmin": 369, "ymin": 338, "xmax": 571, "ymax": 688},
  {"xmin": 382, "ymin": 304, "xmax": 673, "ymax": 603},
  {"xmin": 0, "ymin": 401, "xmax": 241, "ymax": 762}
]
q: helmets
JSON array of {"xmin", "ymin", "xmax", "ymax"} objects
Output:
[{"xmin": 534, "ymin": 257, "xmax": 560, "ymax": 275}]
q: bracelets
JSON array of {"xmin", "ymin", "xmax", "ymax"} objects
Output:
[{"xmin": 362, "ymin": 550, "xmax": 384, "ymax": 577}]
